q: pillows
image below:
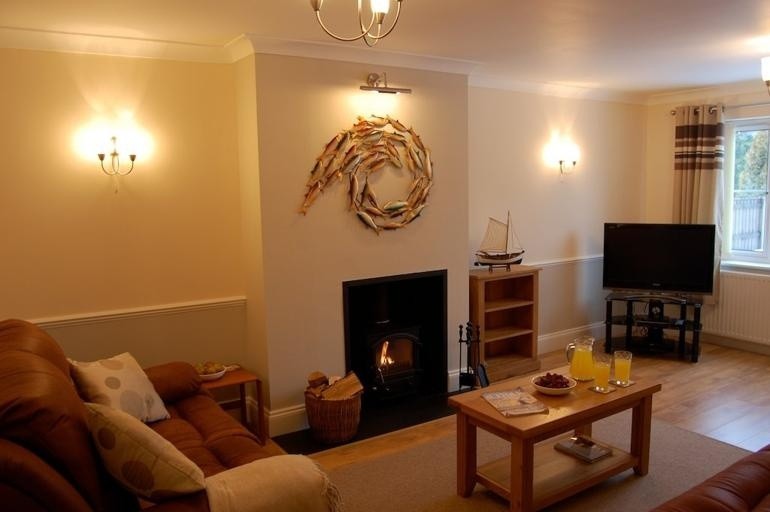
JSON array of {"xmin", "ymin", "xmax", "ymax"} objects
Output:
[
  {"xmin": 80, "ymin": 403, "xmax": 206, "ymax": 494},
  {"xmin": 67, "ymin": 351, "xmax": 171, "ymax": 421}
]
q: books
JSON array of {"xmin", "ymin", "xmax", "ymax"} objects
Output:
[
  {"xmin": 554, "ymin": 434, "xmax": 613, "ymax": 464},
  {"xmin": 480, "ymin": 386, "xmax": 549, "ymax": 417},
  {"xmin": 320, "ymin": 371, "xmax": 364, "ymax": 400}
]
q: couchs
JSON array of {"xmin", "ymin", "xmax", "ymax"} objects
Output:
[
  {"xmin": 2, "ymin": 319, "xmax": 342, "ymax": 512},
  {"xmin": 649, "ymin": 444, "xmax": 770, "ymax": 512}
]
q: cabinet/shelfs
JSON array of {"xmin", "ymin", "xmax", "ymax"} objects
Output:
[
  {"xmin": 469, "ymin": 264, "xmax": 543, "ymax": 383},
  {"xmin": 602, "ymin": 290, "xmax": 704, "ymax": 365}
]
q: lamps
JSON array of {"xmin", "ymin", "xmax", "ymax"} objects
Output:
[
  {"xmin": 359, "ymin": 73, "xmax": 412, "ymax": 96},
  {"xmin": 762, "ymin": 57, "xmax": 770, "ymax": 95},
  {"xmin": 309, "ymin": 0, "xmax": 402, "ymax": 48},
  {"xmin": 97, "ymin": 136, "xmax": 136, "ymax": 176},
  {"xmin": 559, "ymin": 160, "xmax": 577, "ymax": 179}
]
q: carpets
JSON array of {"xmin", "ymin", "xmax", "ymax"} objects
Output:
[{"xmin": 304, "ymin": 408, "xmax": 752, "ymax": 512}]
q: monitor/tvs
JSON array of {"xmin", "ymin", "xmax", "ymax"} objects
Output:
[{"xmin": 604, "ymin": 223, "xmax": 716, "ymax": 303}]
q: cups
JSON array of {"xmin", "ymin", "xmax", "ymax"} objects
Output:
[
  {"xmin": 566, "ymin": 336, "xmax": 596, "ymax": 382},
  {"xmin": 594, "ymin": 354, "xmax": 610, "ymax": 391},
  {"xmin": 615, "ymin": 350, "xmax": 633, "ymax": 385}
]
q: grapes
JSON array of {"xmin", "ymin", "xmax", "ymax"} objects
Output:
[{"xmin": 535, "ymin": 372, "xmax": 569, "ymax": 388}]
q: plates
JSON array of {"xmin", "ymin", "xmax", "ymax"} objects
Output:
[
  {"xmin": 196, "ymin": 365, "xmax": 229, "ymax": 379},
  {"xmin": 532, "ymin": 376, "xmax": 579, "ymax": 395}
]
